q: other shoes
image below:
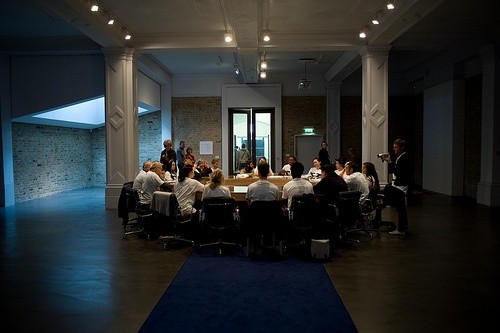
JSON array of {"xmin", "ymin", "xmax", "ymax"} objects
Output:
[{"xmin": 389, "ymin": 230, "xmax": 405, "ymax": 234}]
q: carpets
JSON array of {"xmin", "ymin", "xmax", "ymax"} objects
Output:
[{"xmin": 138, "ymin": 247, "xmax": 361, "ymax": 333}]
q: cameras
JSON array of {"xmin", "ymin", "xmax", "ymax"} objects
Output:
[{"xmin": 378, "ymin": 152, "xmax": 389, "ymax": 161}]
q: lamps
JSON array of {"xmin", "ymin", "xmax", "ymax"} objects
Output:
[
  {"xmin": 106, "ymin": 16, "xmax": 114, "ymax": 24},
  {"xmin": 358, "ymin": 26, "xmax": 367, "ymax": 38},
  {"xmin": 371, "ymin": 12, "xmax": 384, "ymax": 25},
  {"xmin": 387, "ymin": 0, "xmax": 399, "ymax": 10},
  {"xmin": 261, "ymin": 60, "xmax": 267, "ymax": 68},
  {"xmin": 88, "ymin": 0, "xmax": 99, "ymax": 12},
  {"xmin": 263, "ymin": 29, "xmax": 271, "ymax": 42},
  {"xmin": 224, "ymin": 31, "xmax": 232, "ymax": 42},
  {"xmin": 261, "ymin": 69, "xmax": 266, "ymax": 78}
]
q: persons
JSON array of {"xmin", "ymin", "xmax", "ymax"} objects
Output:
[
  {"xmin": 163, "ymin": 159, "xmax": 178, "ymax": 183},
  {"xmin": 178, "ymin": 158, "xmax": 223, "ymax": 176},
  {"xmin": 246, "ymin": 162, "xmax": 279, "ymax": 205},
  {"xmin": 140, "ymin": 162, "xmax": 171, "ymax": 212},
  {"xmin": 386, "ymin": 139, "xmax": 411, "ymax": 235},
  {"xmin": 343, "ymin": 160, "xmax": 370, "ymax": 203},
  {"xmin": 238, "ymin": 143, "xmax": 250, "ymax": 169},
  {"xmin": 314, "ymin": 164, "xmax": 349, "ymax": 217},
  {"xmin": 200, "ymin": 167, "xmax": 232, "ymax": 218},
  {"xmin": 361, "ymin": 162, "xmax": 381, "ymax": 195},
  {"xmin": 241, "ymin": 147, "xmax": 360, "ymax": 177},
  {"xmin": 282, "ymin": 162, "xmax": 314, "ymax": 218},
  {"xmin": 160, "ymin": 140, "xmax": 178, "ymax": 170},
  {"xmin": 173, "ymin": 165, "xmax": 207, "ymax": 219},
  {"xmin": 186, "ymin": 147, "xmax": 196, "ymax": 161},
  {"xmin": 175, "ymin": 141, "xmax": 187, "ymax": 165},
  {"xmin": 319, "ymin": 141, "xmax": 331, "ymax": 166},
  {"xmin": 132, "ymin": 160, "xmax": 156, "ymax": 198}
]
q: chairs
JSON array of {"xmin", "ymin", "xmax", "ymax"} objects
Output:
[{"xmin": 119, "ymin": 182, "xmax": 395, "ymax": 256}]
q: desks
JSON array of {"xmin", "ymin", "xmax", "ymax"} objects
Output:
[{"xmin": 163, "ymin": 175, "xmax": 356, "ymax": 229}]
